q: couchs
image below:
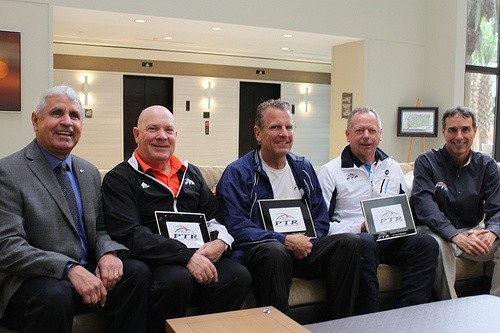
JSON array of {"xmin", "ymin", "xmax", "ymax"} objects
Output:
[{"xmin": 70, "ymin": 161, "xmax": 493, "ymax": 333}]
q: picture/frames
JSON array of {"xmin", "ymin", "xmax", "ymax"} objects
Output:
[{"xmin": 397, "ymin": 107, "xmax": 439, "ymax": 138}]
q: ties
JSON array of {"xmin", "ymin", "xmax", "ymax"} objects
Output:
[{"xmin": 55, "ymin": 162, "xmax": 78, "ymax": 232}]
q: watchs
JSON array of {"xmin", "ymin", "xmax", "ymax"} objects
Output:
[{"xmin": 221, "ymin": 239, "xmax": 230, "ymax": 253}]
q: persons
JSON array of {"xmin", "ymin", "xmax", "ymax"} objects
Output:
[
  {"xmin": 314, "ymin": 105, "xmax": 439, "ymax": 315},
  {"xmin": 216, "ymin": 100, "xmax": 363, "ymax": 326},
  {"xmin": 410, "ymin": 108, "xmax": 500, "ymax": 299},
  {"xmin": 102, "ymin": 104, "xmax": 253, "ymax": 333},
  {"xmin": 0, "ymin": 86, "xmax": 149, "ymax": 333}
]
